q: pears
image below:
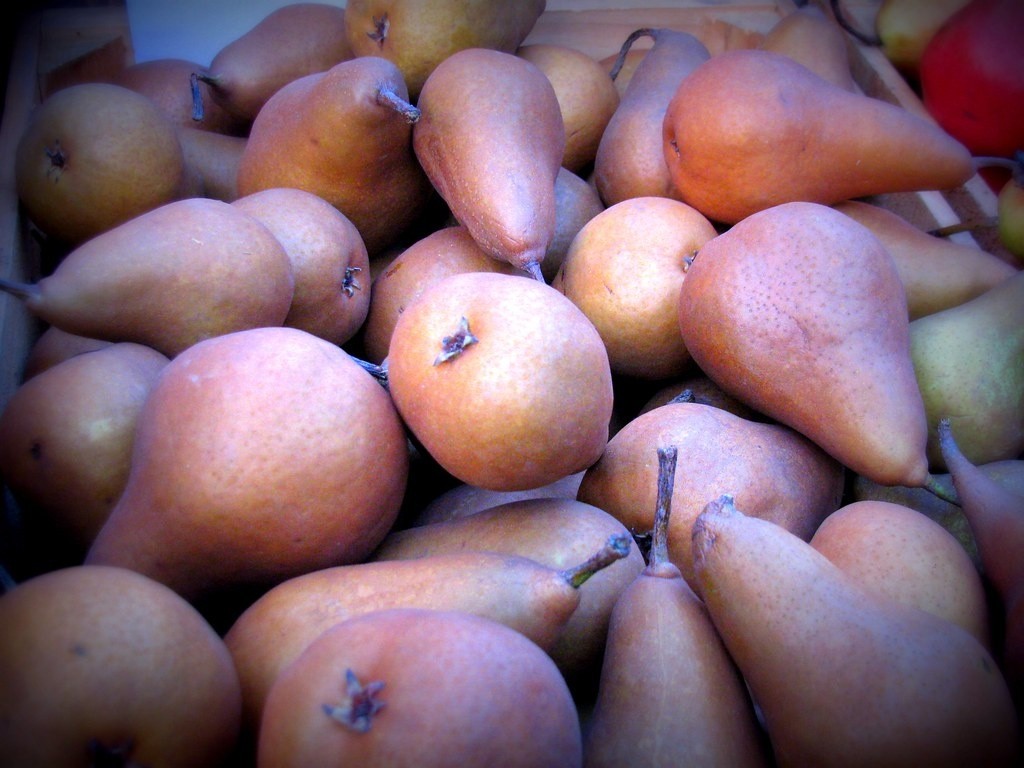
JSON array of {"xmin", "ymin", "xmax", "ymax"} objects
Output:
[{"xmin": 0, "ymin": 0, "xmax": 1024, "ymax": 768}]
